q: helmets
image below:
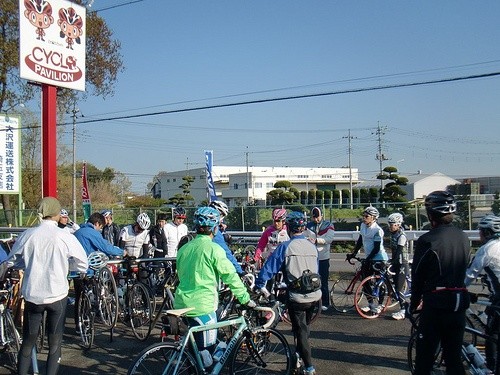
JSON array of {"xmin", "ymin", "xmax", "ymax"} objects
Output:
[
  {"xmin": 425, "ymin": 191, "xmax": 456, "ymax": 214},
  {"xmin": 209, "ymin": 200, "xmax": 228, "ymax": 217},
  {"xmin": 479, "ymin": 214, "xmax": 500, "ymax": 232},
  {"xmin": 102, "ymin": 210, "xmax": 112, "ymax": 218},
  {"xmin": 137, "ymin": 213, "xmax": 151, "ymax": 230},
  {"xmin": 193, "ymin": 207, "xmax": 220, "ymax": 226},
  {"xmin": 60, "ymin": 209, "xmax": 69, "ymax": 218},
  {"xmin": 88, "ymin": 251, "xmax": 109, "ymax": 270},
  {"xmin": 387, "ymin": 213, "xmax": 404, "ymax": 223},
  {"xmin": 362, "ymin": 206, "xmax": 379, "ymax": 220},
  {"xmin": 174, "ymin": 206, "xmax": 186, "ymax": 219},
  {"xmin": 286, "ymin": 212, "xmax": 307, "ymax": 227},
  {"xmin": 272, "ymin": 208, "xmax": 287, "ymax": 221}
]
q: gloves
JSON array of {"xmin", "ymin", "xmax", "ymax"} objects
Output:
[
  {"xmin": 405, "ymin": 303, "xmax": 413, "ymax": 318},
  {"xmin": 346, "ymin": 252, "xmax": 355, "ymax": 259}
]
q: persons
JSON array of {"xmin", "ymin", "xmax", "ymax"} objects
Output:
[
  {"xmin": 172, "ymin": 207, "xmax": 257, "ymax": 358},
  {"xmin": 7, "ymin": 197, "xmax": 88, "ymax": 375},
  {"xmin": 101, "ymin": 210, "xmax": 121, "ymax": 247},
  {"xmin": 347, "ymin": 207, "xmax": 389, "ymax": 314},
  {"xmin": 463, "ymin": 213, "xmax": 500, "ymax": 374},
  {"xmin": 407, "ymin": 190, "xmax": 472, "ymax": 374},
  {"xmin": 386, "ymin": 212, "xmax": 409, "ymax": 321},
  {"xmin": 148, "ymin": 206, "xmax": 188, "ymax": 300},
  {"xmin": 304, "ymin": 206, "xmax": 334, "ymax": 311},
  {"xmin": 72, "ymin": 212, "xmax": 129, "ymax": 334},
  {"xmin": 58, "ymin": 208, "xmax": 80, "ymax": 234},
  {"xmin": 0, "ymin": 245, "xmax": 9, "ymax": 281},
  {"xmin": 115, "ymin": 213, "xmax": 153, "ymax": 323},
  {"xmin": 255, "ymin": 210, "xmax": 323, "ymax": 375},
  {"xmin": 210, "ymin": 200, "xmax": 247, "ymax": 276},
  {"xmin": 254, "ymin": 208, "xmax": 290, "ymax": 293}
]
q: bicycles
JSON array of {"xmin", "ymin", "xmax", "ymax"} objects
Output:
[
  {"xmin": 0, "ymin": 242, "xmax": 324, "ymax": 375},
  {"xmin": 329, "ymin": 253, "xmax": 500, "ymax": 375},
  {"xmin": 126, "ymin": 303, "xmax": 292, "ymax": 375}
]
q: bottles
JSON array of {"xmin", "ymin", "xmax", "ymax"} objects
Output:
[
  {"xmin": 464, "ymin": 342, "xmax": 484, "ymax": 367},
  {"xmin": 117, "ymin": 287, "xmax": 125, "ymax": 303},
  {"xmin": 213, "ymin": 338, "xmax": 227, "ymax": 361},
  {"xmin": 198, "ymin": 345, "xmax": 213, "ymax": 367},
  {"xmin": 477, "ymin": 310, "xmax": 488, "ymax": 325},
  {"xmin": 261, "ymin": 287, "xmax": 271, "ymax": 297}
]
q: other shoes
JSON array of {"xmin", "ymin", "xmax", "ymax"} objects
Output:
[
  {"xmin": 302, "ymin": 367, "xmax": 315, "ymax": 375},
  {"xmin": 322, "ymin": 306, "xmax": 328, "ymax": 311}
]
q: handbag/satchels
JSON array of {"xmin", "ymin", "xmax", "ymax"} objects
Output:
[
  {"xmin": 287, "ymin": 269, "xmax": 321, "ymax": 295},
  {"xmin": 252, "ymin": 301, "xmax": 280, "ymax": 329}
]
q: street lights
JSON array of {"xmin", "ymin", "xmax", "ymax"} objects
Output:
[{"xmin": 69, "ymin": 108, "xmax": 86, "ymax": 223}]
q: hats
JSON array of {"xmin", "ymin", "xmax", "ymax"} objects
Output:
[
  {"xmin": 177, "ymin": 214, "xmax": 186, "ymax": 218},
  {"xmin": 37, "ymin": 197, "xmax": 61, "ymax": 217},
  {"xmin": 311, "ymin": 207, "xmax": 323, "ymax": 217}
]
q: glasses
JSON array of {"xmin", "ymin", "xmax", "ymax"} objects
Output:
[
  {"xmin": 274, "ymin": 218, "xmax": 284, "ymax": 222},
  {"xmin": 176, "ymin": 217, "xmax": 185, "ymax": 219}
]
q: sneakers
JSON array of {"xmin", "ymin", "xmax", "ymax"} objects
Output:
[
  {"xmin": 392, "ymin": 309, "xmax": 406, "ymax": 320},
  {"xmin": 361, "ymin": 306, "xmax": 374, "ymax": 312},
  {"xmin": 372, "ymin": 304, "xmax": 383, "ymax": 313}
]
